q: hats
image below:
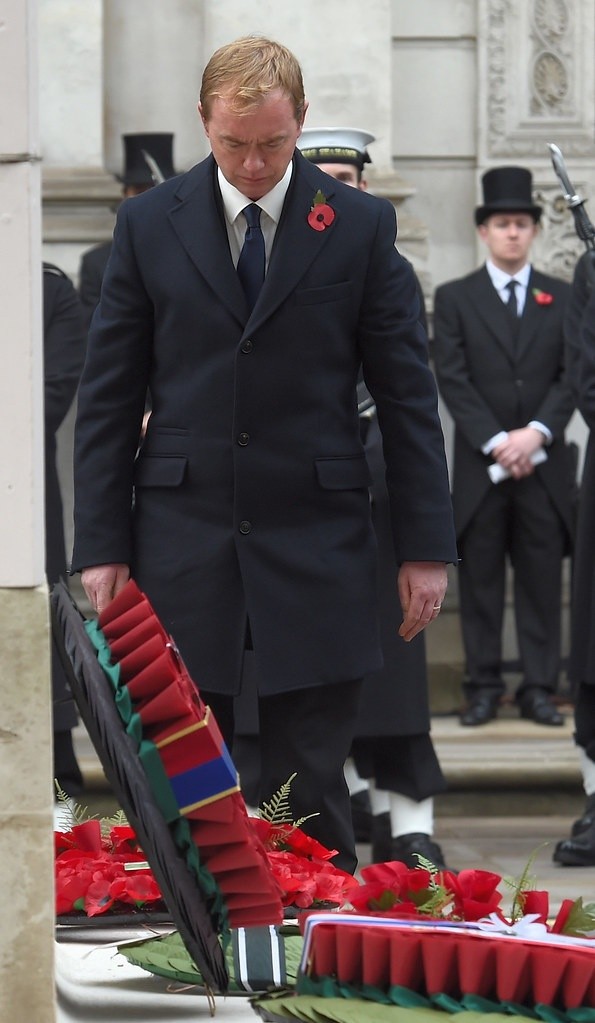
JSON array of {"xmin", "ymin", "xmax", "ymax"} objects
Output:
[
  {"xmin": 113, "ymin": 133, "xmax": 183, "ymax": 186},
  {"xmin": 474, "ymin": 167, "xmax": 543, "ymax": 224},
  {"xmin": 295, "ymin": 128, "xmax": 376, "ymax": 169}
]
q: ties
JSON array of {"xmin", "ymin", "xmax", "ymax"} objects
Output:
[
  {"xmin": 506, "ymin": 280, "xmax": 519, "ymax": 323},
  {"xmin": 237, "ymin": 204, "xmax": 267, "ymax": 311}
]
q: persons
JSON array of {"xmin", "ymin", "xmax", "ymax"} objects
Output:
[
  {"xmin": 76, "ymin": 131, "xmax": 180, "ymax": 343},
  {"xmin": 64, "ymin": 33, "xmax": 456, "ymax": 932},
  {"xmin": 432, "ymin": 164, "xmax": 595, "ymax": 868},
  {"xmin": 42, "ymin": 257, "xmax": 83, "ymax": 796},
  {"xmin": 222, "ymin": 123, "xmax": 445, "ymax": 871}
]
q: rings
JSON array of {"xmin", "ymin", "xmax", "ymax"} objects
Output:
[{"xmin": 434, "ymin": 606, "xmax": 441, "ymax": 610}]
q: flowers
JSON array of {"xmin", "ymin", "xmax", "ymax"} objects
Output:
[
  {"xmin": 532, "ymin": 288, "xmax": 554, "ymax": 307},
  {"xmin": 54, "ymin": 779, "xmax": 594, "ymax": 942},
  {"xmin": 309, "ymin": 189, "xmax": 334, "ymax": 231}
]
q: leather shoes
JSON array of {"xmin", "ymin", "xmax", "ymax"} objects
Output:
[
  {"xmin": 461, "ymin": 698, "xmax": 502, "ymax": 726},
  {"xmin": 522, "ymin": 689, "xmax": 562, "ymax": 725},
  {"xmin": 553, "ymin": 822, "xmax": 595, "ymax": 864}
]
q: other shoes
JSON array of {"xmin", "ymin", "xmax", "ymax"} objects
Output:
[
  {"xmin": 54, "ymin": 730, "xmax": 84, "ymax": 796},
  {"xmin": 392, "ymin": 833, "xmax": 456, "ymax": 875}
]
